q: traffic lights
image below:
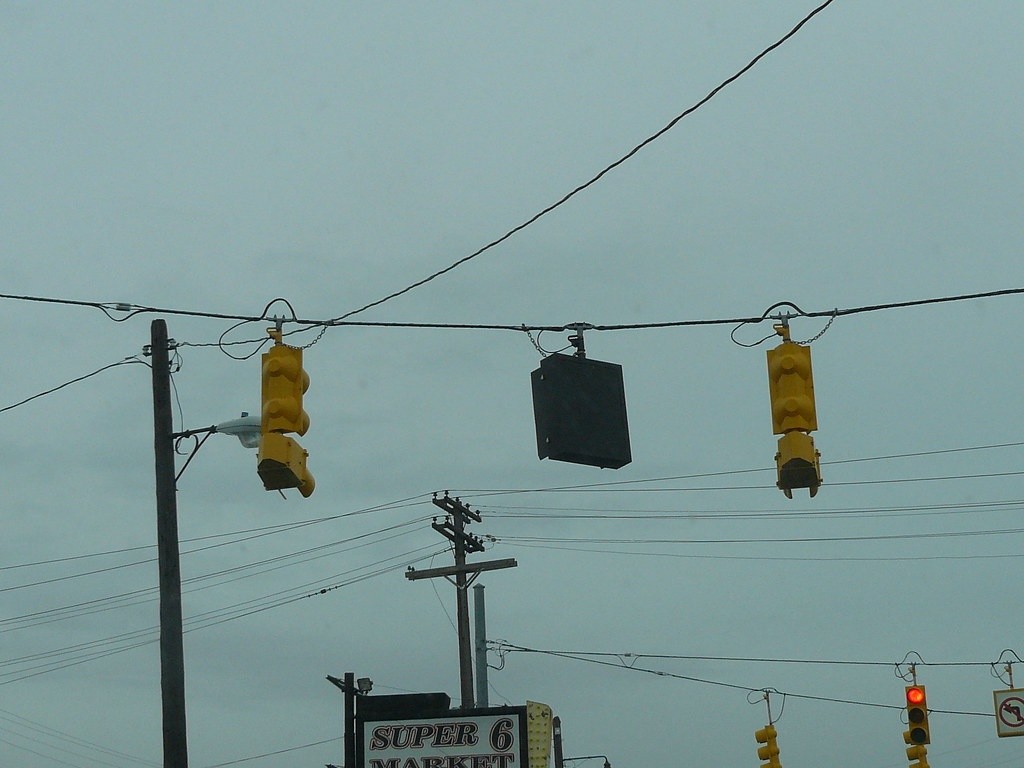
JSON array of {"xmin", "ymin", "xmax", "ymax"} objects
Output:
[
  {"xmin": 257, "ymin": 346, "xmax": 314, "ymax": 498},
  {"xmin": 905, "ymin": 686, "xmax": 930, "ymax": 745},
  {"xmin": 903, "ymin": 731, "xmax": 930, "ymax": 768},
  {"xmin": 755, "ymin": 725, "xmax": 782, "ymax": 768}
]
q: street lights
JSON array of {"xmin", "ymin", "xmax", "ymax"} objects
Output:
[{"xmin": 153, "ymin": 412, "xmax": 262, "ymax": 768}]
